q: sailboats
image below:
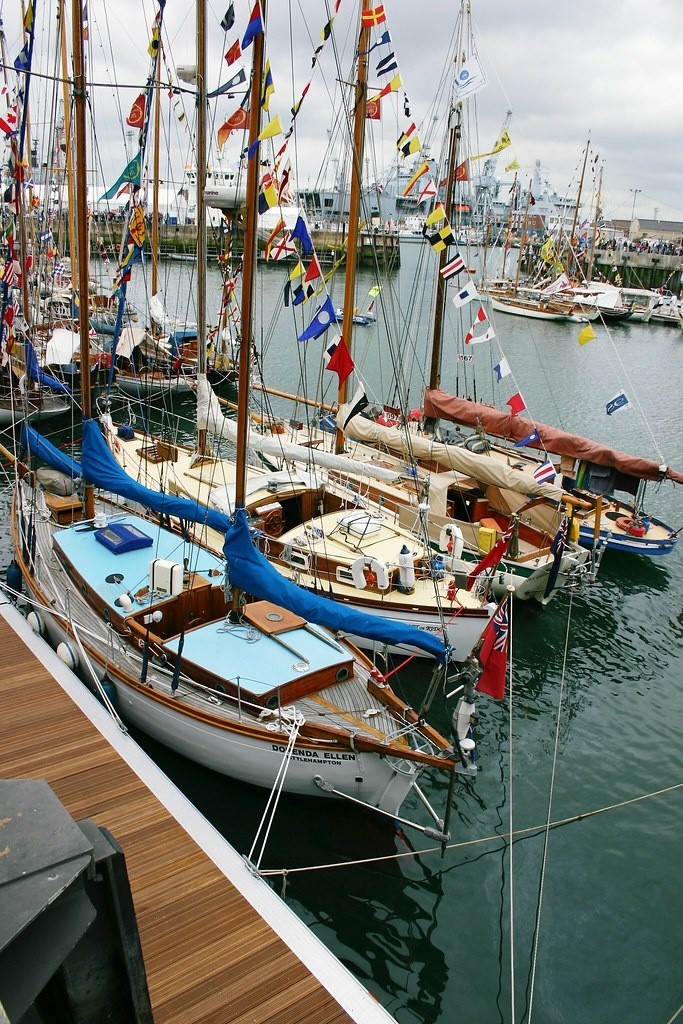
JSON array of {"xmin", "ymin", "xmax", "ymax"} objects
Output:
[{"xmin": 0, "ymin": 2, "xmax": 683, "ymax": 844}]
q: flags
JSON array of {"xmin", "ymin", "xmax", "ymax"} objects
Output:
[
  {"xmin": 267, "ymin": 213, "xmax": 389, "ymax": 394},
  {"xmin": 212, "ymin": 0, "xmax": 307, "ymax": 210},
  {"xmin": 439, "ymin": 252, "xmax": 467, "ymax": 281},
  {"xmin": 452, "ymin": 279, "xmax": 477, "ymax": 309},
  {"xmin": 532, "ymin": 459, "xmax": 556, "ymax": 485},
  {"xmin": 193, "ymin": 207, "xmax": 259, "ymax": 372},
  {"xmin": 97, "ymin": 0, "xmax": 167, "ymax": 327},
  {"xmin": 514, "ymin": 429, "xmax": 540, "ymax": 448},
  {"xmin": 310, "ymin": 4, "xmax": 460, "ymax": 255},
  {"xmin": 462, "ymin": 306, "xmax": 488, "ymax": 345},
  {"xmin": 0, "ymin": 0, "xmax": 65, "ymax": 385},
  {"xmin": 492, "ymin": 356, "xmax": 512, "ymax": 383},
  {"xmin": 505, "ymin": 392, "xmax": 526, "ymax": 416},
  {"xmin": 475, "ymin": 595, "xmax": 510, "ymax": 700},
  {"xmin": 465, "ymin": 527, "xmax": 514, "ymax": 591},
  {"xmin": 453, "ymin": 46, "xmax": 682, "ymax": 417},
  {"xmin": 469, "ymin": 326, "xmax": 496, "ymax": 346},
  {"xmin": 543, "ymin": 515, "xmax": 569, "ymax": 599}
]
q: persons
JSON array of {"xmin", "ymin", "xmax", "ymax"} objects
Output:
[
  {"xmin": 669, "ymin": 293, "xmax": 679, "ymax": 318},
  {"xmin": 655, "ymin": 291, "xmax": 664, "ymax": 314}
]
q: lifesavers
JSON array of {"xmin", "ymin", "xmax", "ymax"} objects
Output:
[
  {"xmin": 351, "ymin": 556, "xmax": 390, "ymax": 590},
  {"xmin": 439, "ymin": 522, "xmax": 465, "ymax": 559}
]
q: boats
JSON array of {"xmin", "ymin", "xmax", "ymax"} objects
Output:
[{"xmin": 297, "ymin": 150, "xmax": 582, "ymax": 243}]
